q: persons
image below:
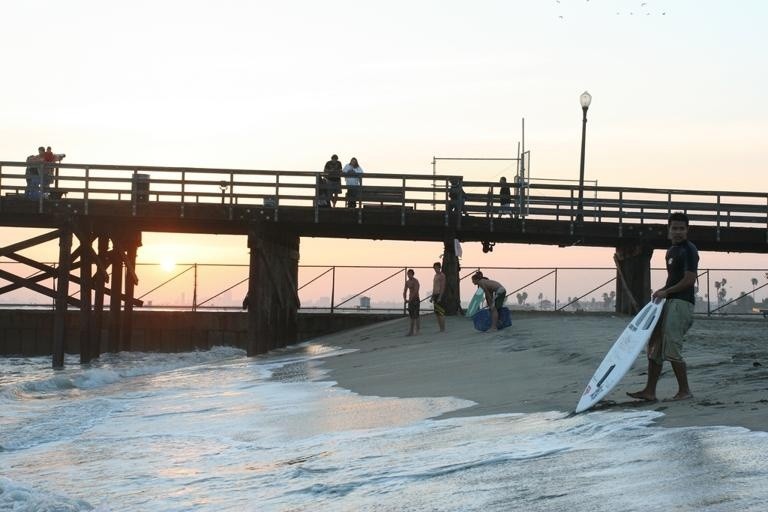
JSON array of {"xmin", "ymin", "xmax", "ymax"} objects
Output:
[
  {"xmin": 472, "ymin": 275, "xmax": 506, "ymax": 332},
  {"xmin": 449, "ymin": 178, "xmax": 469, "ymax": 217},
  {"xmin": 499, "ymin": 177, "xmax": 513, "ymax": 219},
  {"xmin": 26, "ymin": 146, "xmax": 55, "ymax": 198},
  {"xmin": 343, "ymin": 157, "xmax": 363, "ymax": 208},
  {"xmin": 626, "ymin": 214, "xmax": 699, "ymax": 401},
  {"xmin": 403, "ymin": 270, "xmax": 420, "ymax": 336},
  {"xmin": 476, "ymin": 272, "xmax": 491, "ymax": 309},
  {"xmin": 430, "ymin": 263, "xmax": 445, "ymax": 333},
  {"xmin": 324, "ymin": 155, "xmax": 342, "ymax": 208}
]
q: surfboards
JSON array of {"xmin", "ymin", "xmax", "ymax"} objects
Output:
[
  {"xmin": 575, "ymin": 298, "xmax": 667, "ymax": 413},
  {"xmin": 466, "ymin": 287, "xmax": 485, "ymax": 316}
]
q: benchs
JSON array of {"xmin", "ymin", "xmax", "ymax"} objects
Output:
[{"xmin": 343, "ymin": 184, "xmax": 406, "ymax": 210}]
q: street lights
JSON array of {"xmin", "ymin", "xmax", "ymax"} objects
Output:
[{"xmin": 575, "ymin": 91, "xmax": 592, "ymax": 222}]
